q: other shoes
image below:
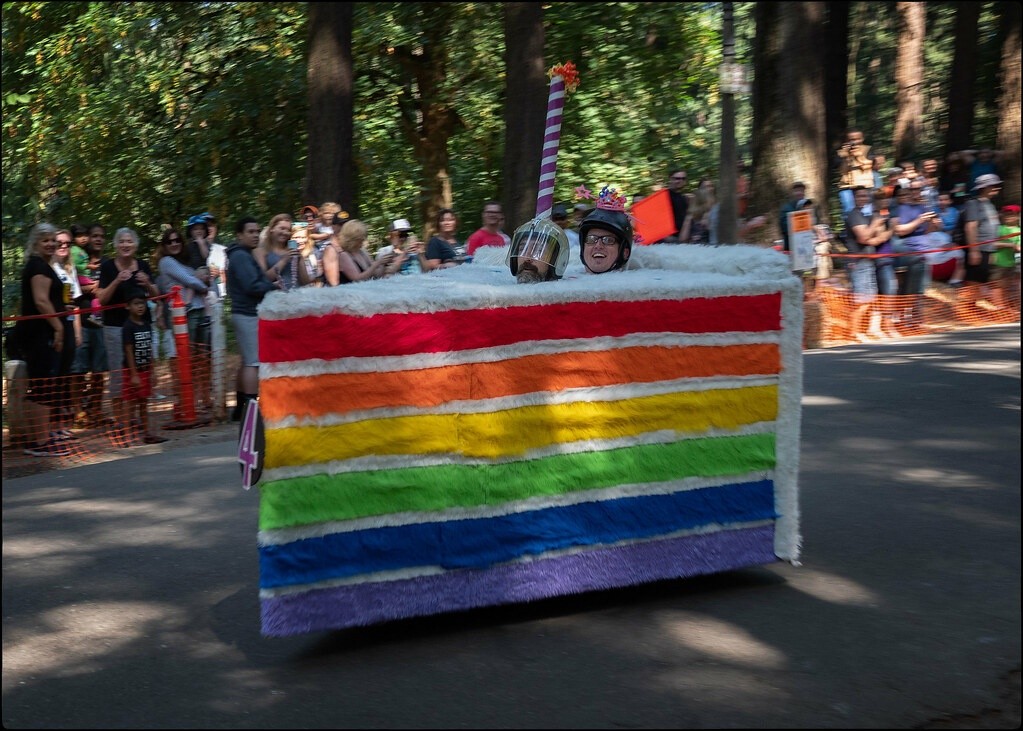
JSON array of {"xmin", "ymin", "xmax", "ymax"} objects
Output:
[
  {"xmin": 48, "ymin": 429, "xmax": 80, "ymax": 440},
  {"xmin": 86, "ymin": 315, "xmax": 103, "ymax": 328},
  {"xmin": 14, "ymin": 395, "xmax": 255, "ymax": 456},
  {"xmin": 840, "ymin": 316, "xmax": 947, "ymax": 345}
]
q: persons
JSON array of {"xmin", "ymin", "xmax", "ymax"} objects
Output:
[
  {"xmin": 0, "ymin": 213, "xmax": 279, "ymax": 460},
  {"xmin": 837, "ymin": 129, "xmax": 1021, "ymax": 340},
  {"xmin": 556, "ymin": 169, "xmax": 765, "ymax": 245},
  {"xmin": 778, "ymin": 182, "xmax": 834, "ymax": 290},
  {"xmin": 577, "ymin": 208, "xmax": 632, "ymax": 273},
  {"xmin": 506, "ymin": 218, "xmax": 570, "ymax": 285},
  {"xmin": 253, "ymin": 199, "xmax": 510, "ymax": 290}
]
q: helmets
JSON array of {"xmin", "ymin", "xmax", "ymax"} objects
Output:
[
  {"xmin": 579, "ymin": 209, "xmax": 634, "ymax": 266},
  {"xmin": 509, "ymin": 219, "xmax": 570, "ymax": 278}
]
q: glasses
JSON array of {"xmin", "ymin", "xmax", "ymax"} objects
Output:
[
  {"xmin": 55, "ymin": 241, "xmax": 72, "ymax": 248},
  {"xmin": 165, "ymin": 237, "xmax": 183, "ymax": 245},
  {"xmin": 582, "ymin": 234, "xmax": 623, "ymax": 246},
  {"xmin": 301, "ymin": 214, "xmax": 318, "ymax": 221}
]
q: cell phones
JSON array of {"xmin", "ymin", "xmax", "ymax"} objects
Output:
[{"xmin": 409, "ymin": 236, "xmax": 416, "ymax": 245}]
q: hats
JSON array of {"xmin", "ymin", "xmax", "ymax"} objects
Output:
[
  {"xmin": 186, "ymin": 215, "xmax": 208, "ymax": 226},
  {"xmin": 551, "ymin": 205, "xmax": 567, "ymax": 217},
  {"xmin": 199, "ymin": 211, "xmax": 216, "ymax": 220},
  {"xmin": 970, "ymin": 173, "xmax": 1001, "ymax": 190},
  {"xmin": 300, "ymin": 205, "xmax": 318, "ymax": 215},
  {"xmin": 383, "ymin": 218, "xmax": 416, "ymax": 240},
  {"xmin": 574, "ymin": 203, "xmax": 589, "ymax": 213},
  {"xmin": 332, "ymin": 210, "xmax": 350, "ymax": 224}
]
